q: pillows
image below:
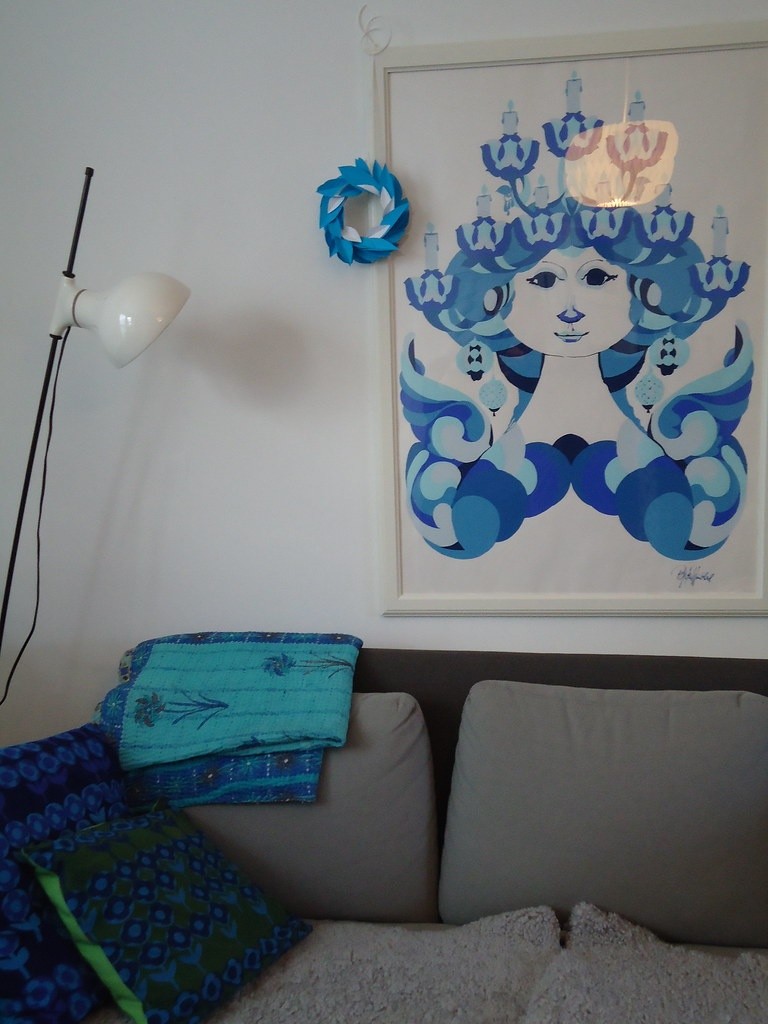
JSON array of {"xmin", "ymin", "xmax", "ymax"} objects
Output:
[
  {"xmin": 0, "ymin": 723, "xmax": 313, "ymax": 1024},
  {"xmin": 437, "ymin": 680, "xmax": 768, "ymax": 950},
  {"xmin": 181, "ymin": 693, "xmax": 438, "ymax": 923}
]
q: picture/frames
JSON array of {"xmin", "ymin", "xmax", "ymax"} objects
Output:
[{"xmin": 370, "ymin": 18, "xmax": 768, "ymax": 619}]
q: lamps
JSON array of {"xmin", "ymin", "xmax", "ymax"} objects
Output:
[{"xmin": 0, "ymin": 166, "xmax": 187, "ymax": 704}]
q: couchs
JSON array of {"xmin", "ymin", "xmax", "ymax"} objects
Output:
[{"xmin": 0, "ymin": 631, "xmax": 768, "ymax": 1024}]
